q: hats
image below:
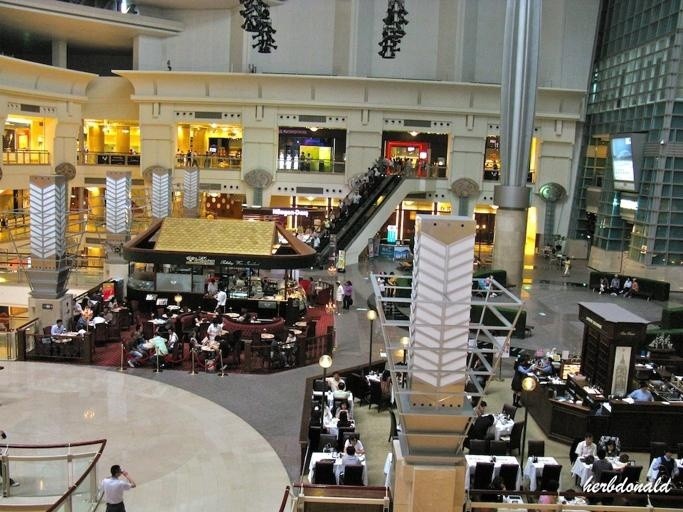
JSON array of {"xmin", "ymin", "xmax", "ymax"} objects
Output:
[{"xmin": 336, "ymin": 281, "xmax": 340, "ymax": 283}]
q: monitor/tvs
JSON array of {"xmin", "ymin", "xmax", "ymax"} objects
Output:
[{"xmin": 607, "ymin": 133, "xmax": 647, "ymax": 196}]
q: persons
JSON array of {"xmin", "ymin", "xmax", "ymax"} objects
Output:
[
  {"xmin": 335, "ymin": 280, "xmax": 345, "ymax": 316},
  {"xmin": 306, "ymin": 153, "xmax": 312, "ymax": 170},
  {"xmin": 208, "ymin": 279, "xmax": 218, "ymax": 295},
  {"xmin": 377, "ymin": 272, "xmax": 397, "ymax": 305},
  {"xmin": 0, "ymin": 430, "xmax": 20, "ymax": 488},
  {"xmin": 510, "ymin": 356, "xmax": 534, "ymax": 407},
  {"xmin": 300, "ymin": 151, "xmax": 305, "ymax": 170},
  {"xmin": 343, "ymin": 280, "xmax": 353, "ymax": 310},
  {"xmin": 99, "ymin": 464, "xmax": 136, "ymax": 512},
  {"xmin": 471, "ymin": 358, "xmax": 489, "ymax": 405},
  {"xmin": 535, "ymin": 357, "xmax": 557, "ymax": 376},
  {"xmin": 177, "ymin": 148, "xmax": 216, "ymax": 168},
  {"xmin": 268, "ymin": 330, "xmax": 297, "ymax": 368},
  {"xmin": 299, "ymin": 276, "xmax": 318, "ymax": 309},
  {"xmin": 316, "ymin": 370, "xmax": 366, "ymax": 476},
  {"xmin": 466, "ymin": 398, "xmax": 680, "ymax": 512},
  {"xmin": 51, "ymin": 319, "xmax": 65, "ymax": 335},
  {"xmin": 598, "ymin": 274, "xmax": 639, "ymax": 299},
  {"xmin": 76, "ymin": 285, "xmax": 232, "ymax": 371},
  {"xmin": 562, "ymin": 257, "xmax": 571, "ymax": 277},
  {"xmin": 626, "ymin": 381, "xmax": 655, "ymax": 402},
  {"xmin": 311, "ymin": 156, "xmax": 440, "ymax": 249}
]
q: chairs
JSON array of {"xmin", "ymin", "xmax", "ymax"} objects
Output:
[
  {"xmin": 461, "ymin": 370, "xmax": 682, "ymax": 508},
  {"xmin": 34, "ymin": 291, "xmax": 300, "ymax": 376},
  {"xmin": 309, "ymin": 356, "xmax": 394, "ymax": 485}
]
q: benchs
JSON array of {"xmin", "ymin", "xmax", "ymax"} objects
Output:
[{"xmin": 589, "ymin": 271, "xmax": 670, "ymax": 302}]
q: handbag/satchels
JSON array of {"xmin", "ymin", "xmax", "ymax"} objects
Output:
[{"xmin": 349, "ymin": 298, "xmax": 352, "ymax": 305}]
